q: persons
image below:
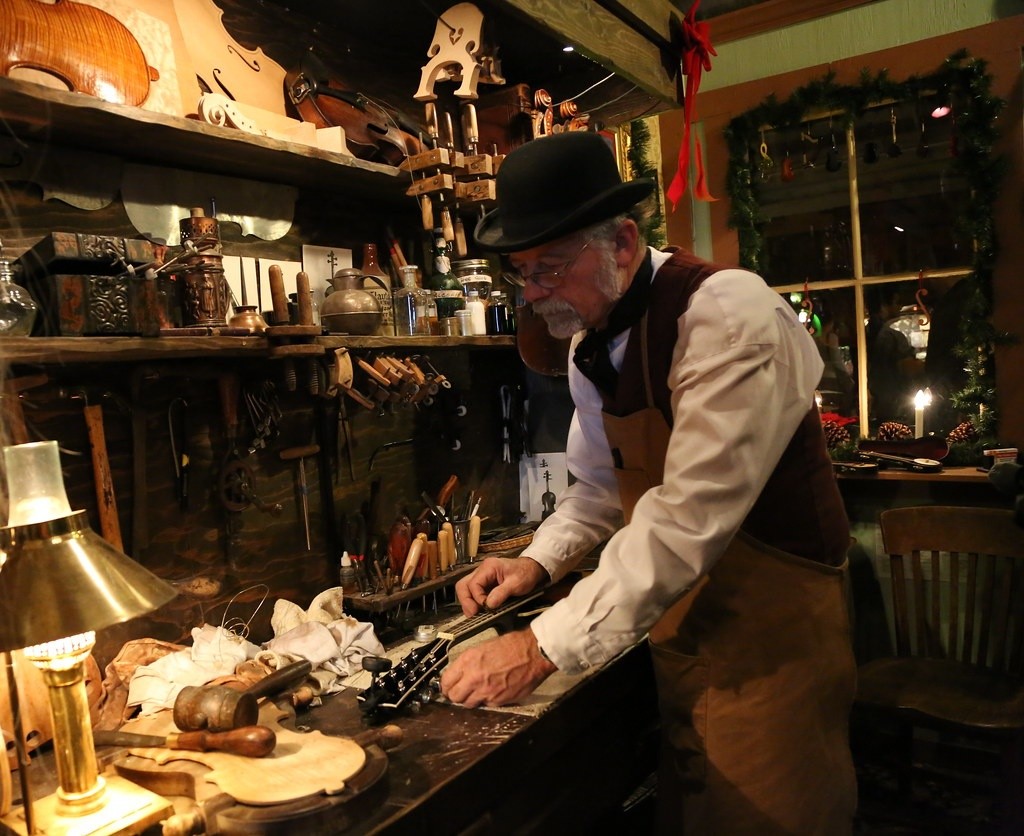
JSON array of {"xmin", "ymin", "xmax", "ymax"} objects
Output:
[
  {"xmin": 439, "ymin": 129, "xmax": 856, "ymax": 836},
  {"xmin": 849, "ymin": 267, "xmax": 958, "ymax": 426}
]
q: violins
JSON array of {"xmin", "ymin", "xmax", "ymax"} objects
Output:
[
  {"xmin": 285, "ymin": 62, "xmax": 455, "ymax": 166},
  {"xmin": 115, "ymin": 708, "xmax": 403, "ymax": 836},
  {"xmin": 513, "ymin": 87, "xmax": 605, "ymax": 377}
]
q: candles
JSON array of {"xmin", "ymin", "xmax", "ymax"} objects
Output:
[{"xmin": 914, "ymin": 390, "xmax": 927, "ymax": 439}]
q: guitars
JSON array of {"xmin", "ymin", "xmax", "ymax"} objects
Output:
[
  {"xmin": 851, "ymin": 446, "xmax": 943, "ymax": 473},
  {"xmin": 829, "ymin": 457, "xmax": 880, "ymax": 475},
  {"xmin": 355, "ymin": 535, "xmax": 627, "ymax": 724}
]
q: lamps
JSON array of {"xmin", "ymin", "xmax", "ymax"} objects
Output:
[
  {"xmin": 885, "ymin": 305, "xmax": 932, "ymax": 360},
  {"xmin": 0, "ymin": 440, "xmax": 178, "ymax": 836}
]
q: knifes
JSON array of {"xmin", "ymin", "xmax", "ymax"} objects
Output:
[{"xmin": 92, "ymin": 726, "xmax": 277, "ymax": 757}]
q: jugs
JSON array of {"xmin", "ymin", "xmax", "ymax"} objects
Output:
[{"xmin": 321, "ymin": 268, "xmax": 392, "ymax": 336}]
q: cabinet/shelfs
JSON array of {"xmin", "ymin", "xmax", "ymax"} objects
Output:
[{"xmin": 0, "ymin": 0, "xmax": 698, "ymax": 836}]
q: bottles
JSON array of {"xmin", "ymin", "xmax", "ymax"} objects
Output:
[
  {"xmin": 820, "ymin": 246, "xmax": 840, "ymax": 279},
  {"xmin": 499, "ymin": 293, "xmax": 515, "ymax": 334},
  {"xmin": 362, "ymin": 244, "xmax": 394, "ymax": 336},
  {"xmin": 465, "ymin": 291, "xmax": 487, "ymax": 335},
  {"xmin": 423, "ymin": 290, "xmax": 438, "ymax": 335},
  {"xmin": 487, "ymin": 291, "xmax": 508, "ymax": 335},
  {"xmin": 309, "ymin": 290, "xmax": 319, "ymax": 325},
  {"xmin": 395, "ymin": 266, "xmax": 430, "ymax": 336},
  {"xmin": 423, "ymin": 229, "xmax": 465, "ymax": 324}
]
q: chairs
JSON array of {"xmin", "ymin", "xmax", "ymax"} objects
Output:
[{"xmin": 852, "ymin": 505, "xmax": 1024, "ymax": 836}]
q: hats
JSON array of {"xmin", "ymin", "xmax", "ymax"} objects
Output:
[{"xmin": 474, "ymin": 131, "xmax": 655, "ymax": 252}]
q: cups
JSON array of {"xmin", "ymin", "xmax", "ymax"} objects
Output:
[{"xmin": 438, "ymin": 517, "xmax": 469, "ymax": 565}]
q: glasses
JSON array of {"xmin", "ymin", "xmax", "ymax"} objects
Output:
[{"xmin": 502, "ymin": 236, "xmax": 595, "ymax": 290}]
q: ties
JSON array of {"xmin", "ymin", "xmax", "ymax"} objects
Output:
[{"xmin": 572, "ymin": 247, "xmax": 654, "ymax": 416}]
